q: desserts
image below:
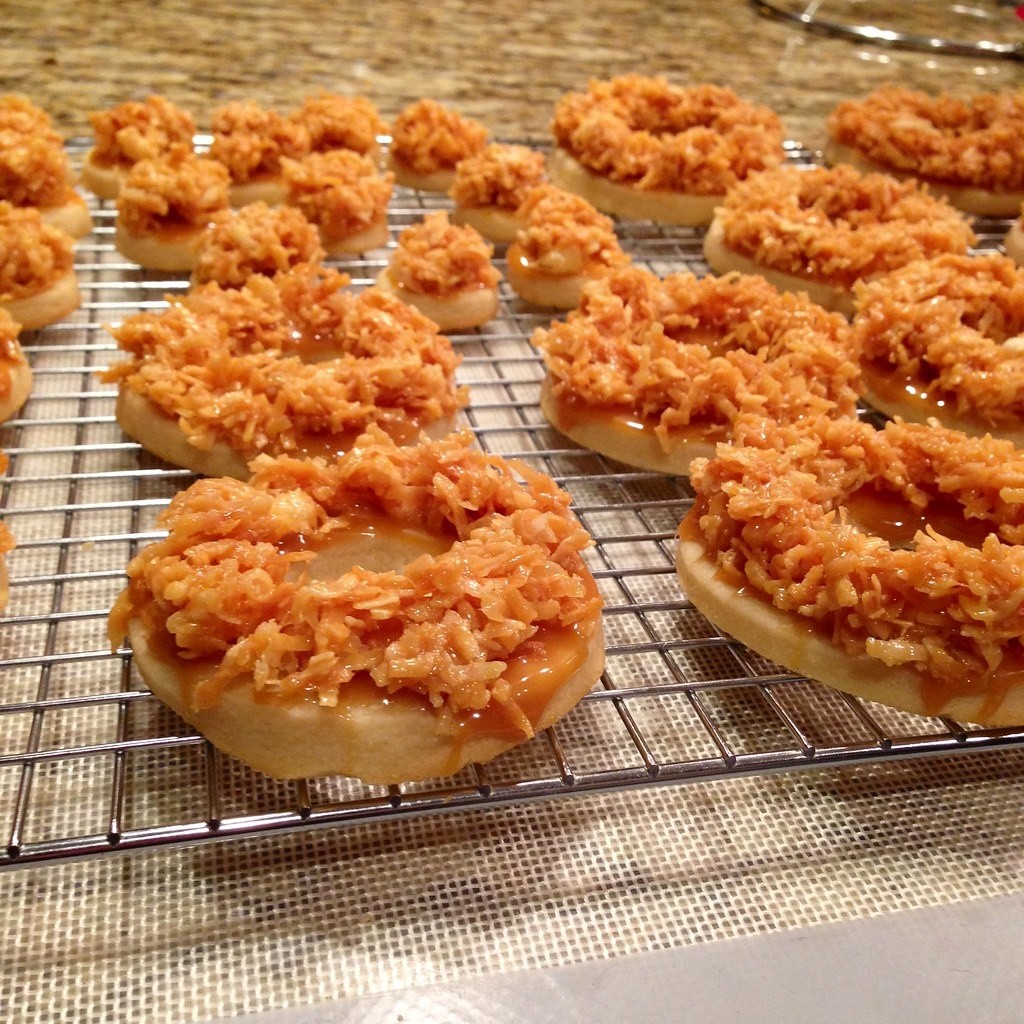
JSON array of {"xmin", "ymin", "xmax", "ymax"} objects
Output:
[{"xmin": 1, "ymin": 73, "xmax": 1024, "ymax": 785}]
